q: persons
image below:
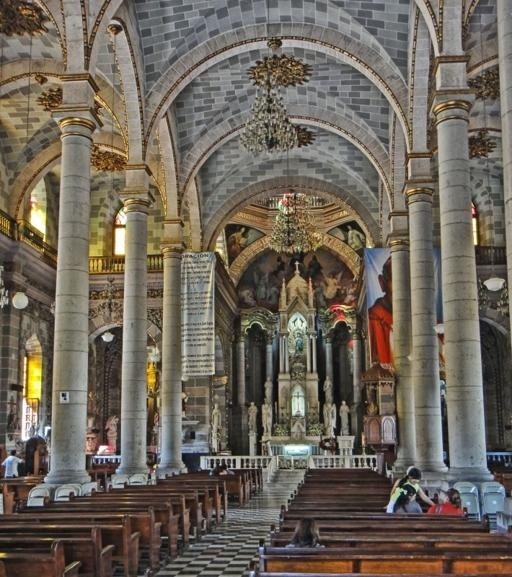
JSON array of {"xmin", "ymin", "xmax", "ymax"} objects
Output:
[
  {"xmin": 307, "ymin": 254, "xmax": 357, "ymax": 307},
  {"xmin": 294, "ymin": 334, "xmax": 303, "ymax": 356},
  {"xmin": 228, "ymin": 225, "xmax": 248, "ymax": 253},
  {"xmin": 214, "ymin": 462, "xmax": 232, "ymax": 477},
  {"xmin": 259, "ymin": 399, "xmax": 275, "ymax": 435},
  {"xmin": 247, "ymin": 402, "xmax": 257, "ymax": 431},
  {"xmin": 105, "ymin": 414, "xmax": 119, "ymax": 436},
  {"xmin": 322, "ymin": 376, "xmax": 335, "ymax": 434},
  {"xmin": 2, "ymin": 448, "xmax": 25, "ymax": 482},
  {"xmin": 385, "ymin": 468, "xmax": 463, "ymax": 519},
  {"xmin": 241, "ymin": 272, "xmax": 279, "ymax": 308},
  {"xmin": 340, "ymin": 400, "xmax": 350, "ymax": 436},
  {"xmin": 346, "ymin": 222, "xmax": 367, "ymax": 251},
  {"xmin": 288, "ymin": 517, "xmax": 322, "ymax": 550},
  {"xmin": 212, "ymin": 403, "xmax": 221, "ymax": 426}
]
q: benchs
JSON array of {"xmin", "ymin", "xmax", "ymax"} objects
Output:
[
  {"xmin": 0, "ymin": 454, "xmax": 262, "ymax": 577},
  {"xmin": 259, "ymin": 465, "xmax": 512, "ymax": 577}
]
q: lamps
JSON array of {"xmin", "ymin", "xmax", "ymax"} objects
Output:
[
  {"xmin": 10, "ymin": 18, "xmax": 34, "ymax": 309},
  {"xmin": 269, "ymin": 186, "xmax": 325, "ymax": 255},
  {"xmin": 464, "ymin": 2, "xmax": 509, "ymax": 294},
  {"xmin": 240, "ymin": 38, "xmax": 317, "ymax": 157},
  {"xmin": 101, "ymin": 327, "xmax": 116, "ymax": 342}
]
